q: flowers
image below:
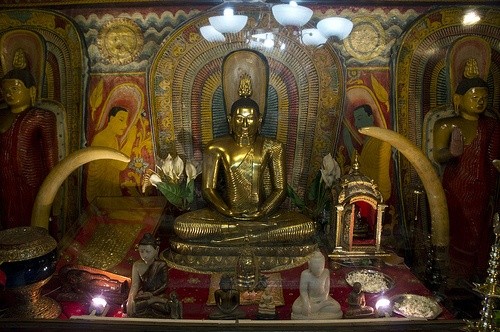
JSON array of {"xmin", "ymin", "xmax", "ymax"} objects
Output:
[
  {"xmin": 286, "ymin": 152, "xmax": 340, "ymax": 219},
  {"xmin": 149, "ymin": 154, "xmax": 197, "ymax": 213}
]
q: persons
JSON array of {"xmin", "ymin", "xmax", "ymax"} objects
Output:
[
  {"xmin": 173, "ymin": 97, "xmax": 316, "ymax": 245},
  {"xmin": 433, "ymin": 79, "xmax": 500, "ymax": 288},
  {"xmin": 0, "ymin": 68, "xmax": 58, "ymax": 230},
  {"xmin": 121, "ymin": 232, "xmax": 171, "ymax": 316},
  {"xmin": 207, "ymin": 276, "xmax": 244, "ymax": 318},
  {"xmin": 259, "ymin": 288, "xmax": 275, "ymax": 309},
  {"xmin": 291, "ymin": 252, "xmax": 341, "ymax": 315},
  {"xmin": 346, "ymin": 282, "xmax": 373, "ymax": 316}
]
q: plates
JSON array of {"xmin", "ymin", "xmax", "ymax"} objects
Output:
[
  {"xmin": 389, "ymin": 294, "xmax": 443, "ymax": 319},
  {"xmin": 345, "ymin": 269, "xmax": 396, "ymax": 293}
]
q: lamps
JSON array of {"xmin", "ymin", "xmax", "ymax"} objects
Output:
[{"xmin": 199, "ymin": 0, "xmax": 354, "ymax": 51}]
